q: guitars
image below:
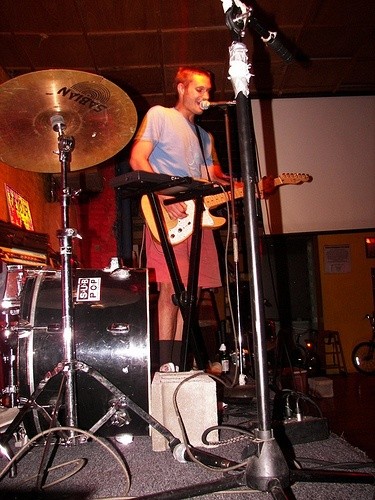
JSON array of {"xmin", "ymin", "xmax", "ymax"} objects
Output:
[{"xmin": 141, "ymin": 172, "xmax": 313, "ymax": 245}]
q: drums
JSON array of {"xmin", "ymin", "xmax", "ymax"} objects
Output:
[
  {"xmin": 0, "ymin": 264, "xmax": 30, "ymax": 318},
  {"xmin": 17, "ymin": 267, "xmax": 153, "ymax": 438},
  {"xmin": 2, "ymin": 325, "xmax": 32, "ymax": 391}
]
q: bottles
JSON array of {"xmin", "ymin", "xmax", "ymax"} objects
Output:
[{"xmin": 220, "ymin": 343, "xmax": 230, "ymax": 374}]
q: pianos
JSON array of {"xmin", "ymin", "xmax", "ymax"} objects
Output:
[{"xmin": 111, "ymin": 170, "xmax": 225, "ymax": 196}]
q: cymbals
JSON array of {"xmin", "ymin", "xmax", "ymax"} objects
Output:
[{"xmin": 0, "ymin": 69, "xmax": 137, "ymax": 175}]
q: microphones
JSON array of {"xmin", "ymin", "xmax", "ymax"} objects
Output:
[{"xmin": 199, "ymin": 100, "xmax": 236, "ymax": 110}]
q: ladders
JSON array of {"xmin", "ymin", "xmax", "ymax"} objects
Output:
[{"xmin": 312, "ymin": 330, "xmax": 347, "ymax": 375}]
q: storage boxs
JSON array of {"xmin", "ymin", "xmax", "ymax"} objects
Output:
[
  {"xmin": 307, "ymin": 377, "xmax": 334, "ymax": 398},
  {"xmin": 283, "ymin": 367, "xmax": 308, "ymax": 394}
]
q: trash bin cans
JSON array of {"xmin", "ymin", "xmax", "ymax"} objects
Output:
[{"xmin": 286, "ymin": 367, "xmax": 308, "ymax": 394}]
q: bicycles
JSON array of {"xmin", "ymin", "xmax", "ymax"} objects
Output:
[{"xmin": 351, "ymin": 314, "xmax": 375, "ymax": 376}]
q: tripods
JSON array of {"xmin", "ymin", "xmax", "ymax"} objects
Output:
[
  {"xmin": 0, "ymin": 116, "xmax": 182, "ymax": 500},
  {"xmin": 133, "ymin": 0, "xmax": 375, "ymax": 500}
]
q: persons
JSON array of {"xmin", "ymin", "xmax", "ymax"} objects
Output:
[{"xmin": 129, "ymin": 66, "xmax": 274, "ymax": 375}]
